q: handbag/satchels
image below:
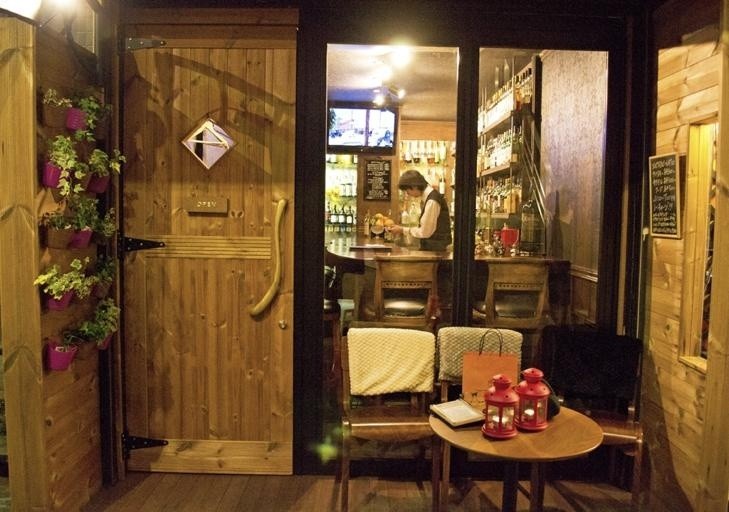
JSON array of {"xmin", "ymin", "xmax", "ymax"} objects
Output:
[{"xmin": 462, "ymin": 327, "xmax": 519, "ymax": 409}]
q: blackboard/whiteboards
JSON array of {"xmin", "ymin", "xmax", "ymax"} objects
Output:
[
  {"xmin": 363, "ymin": 158, "xmax": 391, "ymax": 201},
  {"xmin": 648, "ymin": 152, "xmax": 681, "ymax": 239}
]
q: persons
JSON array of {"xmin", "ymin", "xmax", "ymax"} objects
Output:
[{"xmin": 387, "ymin": 169, "xmax": 452, "ymax": 253}]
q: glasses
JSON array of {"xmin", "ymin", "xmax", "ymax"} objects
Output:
[{"xmin": 459, "ymin": 388, "xmax": 489, "ymax": 406}]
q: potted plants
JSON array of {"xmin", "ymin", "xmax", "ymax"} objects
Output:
[{"xmin": 31, "ymin": 85, "xmax": 126, "ymax": 372}]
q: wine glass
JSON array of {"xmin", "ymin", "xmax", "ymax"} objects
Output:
[{"xmin": 370, "ymin": 225, "xmax": 385, "ymax": 240}]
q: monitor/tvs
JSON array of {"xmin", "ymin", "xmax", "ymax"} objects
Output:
[{"xmin": 327, "ymin": 100, "xmax": 399, "ymax": 155}]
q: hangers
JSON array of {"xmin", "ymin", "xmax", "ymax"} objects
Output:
[{"xmin": 189, "ymin": 120, "xmax": 230, "ymax": 150}]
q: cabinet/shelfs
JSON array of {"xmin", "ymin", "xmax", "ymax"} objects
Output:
[
  {"xmin": 477, "ymin": 108, "xmax": 534, "ymax": 220},
  {"xmin": 325, "ymin": 154, "xmax": 358, "ymax": 232}
]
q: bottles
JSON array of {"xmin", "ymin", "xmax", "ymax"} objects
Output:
[
  {"xmin": 400, "ymin": 65, "xmax": 534, "ymax": 256},
  {"xmin": 324, "ymin": 174, "xmax": 357, "ymax": 238},
  {"xmin": 403, "ymin": 138, "xmax": 450, "ymax": 199},
  {"xmin": 383, "ymin": 209, "xmax": 396, "ymax": 242},
  {"xmin": 363, "ymin": 209, "xmax": 373, "ymax": 238}
]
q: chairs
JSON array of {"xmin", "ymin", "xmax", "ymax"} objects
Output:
[
  {"xmin": 534, "ymin": 325, "xmax": 644, "ymax": 512},
  {"xmin": 437, "ymin": 327, "xmax": 523, "ymax": 405},
  {"xmin": 334, "ymin": 325, "xmax": 443, "ymax": 511},
  {"xmin": 373, "ymin": 256, "xmax": 549, "ymax": 324}
]
